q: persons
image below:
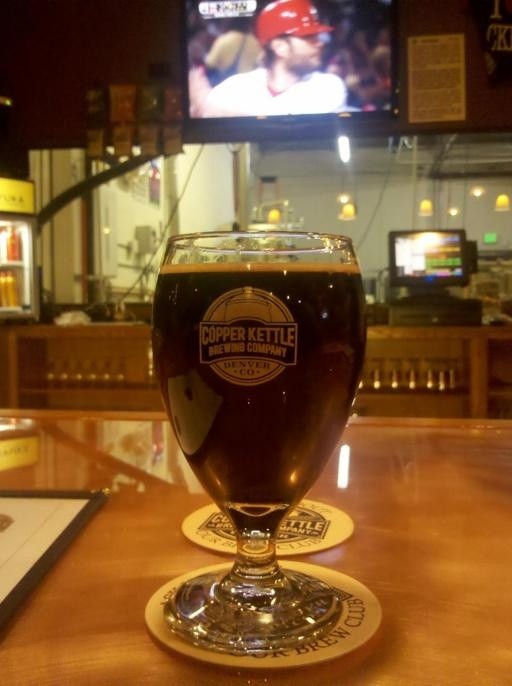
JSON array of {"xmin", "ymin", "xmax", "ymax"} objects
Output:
[{"xmin": 185, "ymin": 1, "xmax": 393, "ymax": 118}]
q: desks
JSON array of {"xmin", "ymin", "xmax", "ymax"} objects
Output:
[{"xmin": 0, "ymin": 402, "xmax": 511, "ymax": 685}]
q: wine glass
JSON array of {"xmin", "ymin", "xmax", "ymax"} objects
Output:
[{"xmin": 143, "ymin": 230, "xmax": 383, "ymax": 670}]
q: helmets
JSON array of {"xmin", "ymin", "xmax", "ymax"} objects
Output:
[{"xmin": 257, "ymin": 0, "xmax": 334, "ymax": 43}]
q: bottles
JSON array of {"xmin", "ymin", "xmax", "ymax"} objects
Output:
[{"xmin": 0, "ymin": 224, "xmax": 23, "ymax": 308}]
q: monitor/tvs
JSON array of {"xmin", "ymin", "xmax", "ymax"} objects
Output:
[
  {"xmin": 179, "ymin": 0, "xmax": 401, "ymax": 142},
  {"xmin": 389, "ymin": 228, "xmax": 469, "ymax": 306}
]
